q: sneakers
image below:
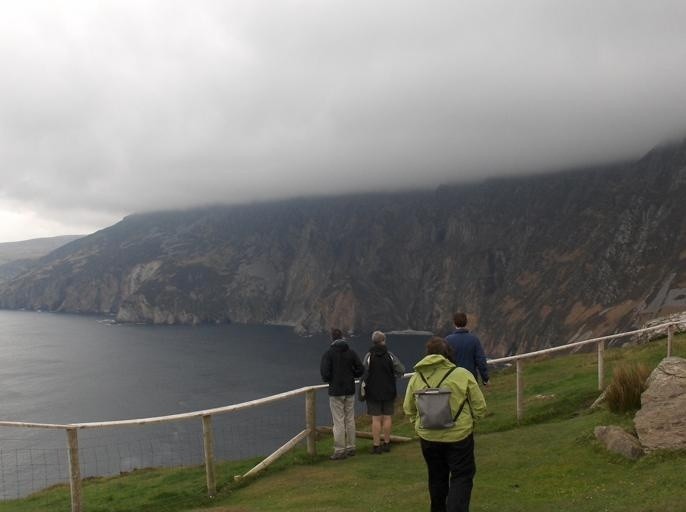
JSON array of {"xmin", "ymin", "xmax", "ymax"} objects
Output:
[
  {"xmin": 369, "ymin": 441, "xmax": 392, "ymax": 454},
  {"xmin": 329, "ymin": 449, "xmax": 357, "ymax": 460}
]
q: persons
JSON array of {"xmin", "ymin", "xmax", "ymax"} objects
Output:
[
  {"xmin": 444, "ymin": 312, "xmax": 492, "ymax": 388},
  {"xmin": 321, "ymin": 328, "xmax": 364, "ymax": 459},
  {"xmin": 402, "ymin": 338, "xmax": 488, "ymax": 512},
  {"xmin": 358, "ymin": 330, "xmax": 405, "ymax": 454}
]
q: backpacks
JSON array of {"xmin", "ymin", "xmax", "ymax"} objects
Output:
[{"xmin": 415, "ymin": 366, "xmax": 467, "ymax": 430}]
